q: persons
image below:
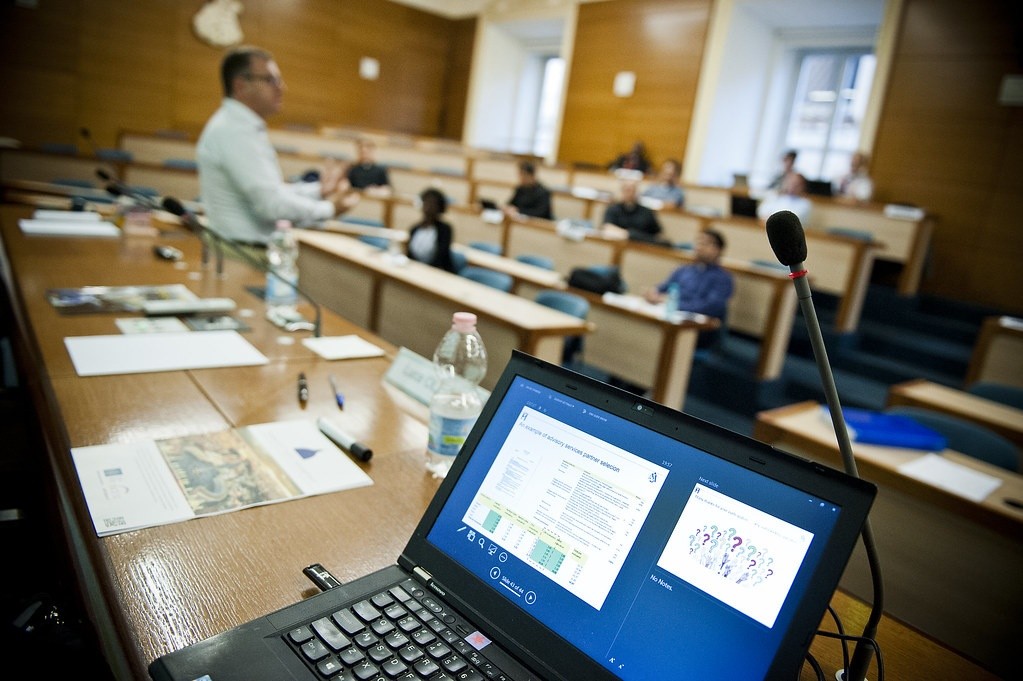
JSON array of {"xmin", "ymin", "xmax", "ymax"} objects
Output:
[
  {"xmin": 405, "ymin": 187, "xmax": 457, "ymax": 273},
  {"xmin": 651, "ymin": 229, "xmax": 735, "ymax": 351},
  {"xmin": 501, "ymin": 160, "xmax": 551, "ymax": 220},
  {"xmin": 637, "ymin": 159, "xmax": 684, "ymax": 210},
  {"xmin": 835, "ymin": 152, "xmax": 873, "ymax": 200},
  {"xmin": 607, "ymin": 142, "xmax": 647, "ymax": 184},
  {"xmin": 337, "ymin": 134, "xmax": 394, "ymax": 200},
  {"xmin": 197, "ymin": 47, "xmax": 361, "ymax": 246},
  {"xmin": 757, "ymin": 173, "xmax": 813, "ymax": 230},
  {"xmin": 598, "ymin": 180, "xmax": 662, "ymax": 244},
  {"xmin": 766, "ymin": 149, "xmax": 797, "ymax": 190}
]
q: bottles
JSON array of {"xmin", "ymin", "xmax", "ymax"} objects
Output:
[
  {"xmin": 263, "ymin": 219, "xmax": 298, "ymax": 310},
  {"xmin": 667, "ymin": 283, "xmax": 680, "ymax": 313},
  {"xmin": 425, "ymin": 311, "xmax": 488, "ymax": 478}
]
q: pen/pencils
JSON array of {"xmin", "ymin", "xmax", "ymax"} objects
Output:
[
  {"xmin": 297, "ymin": 371, "xmax": 311, "ymax": 415},
  {"xmin": 319, "ymin": 416, "xmax": 373, "ymax": 464},
  {"xmin": 328, "ymin": 376, "xmax": 345, "ymax": 407}
]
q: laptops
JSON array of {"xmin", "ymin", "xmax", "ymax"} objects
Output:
[{"xmin": 147, "ymin": 345, "xmax": 878, "ymax": 681}]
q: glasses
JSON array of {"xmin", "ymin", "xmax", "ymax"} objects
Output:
[{"xmin": 242, "ymin": 73, "xmax": 283, "ymax": 83}]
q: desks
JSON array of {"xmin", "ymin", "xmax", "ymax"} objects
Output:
[{"xmin": 0, "ymin": 123, "xmax": 1023, "ymax": 681}]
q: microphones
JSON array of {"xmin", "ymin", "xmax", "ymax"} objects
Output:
[
  {"xmin": 78, "ymin": 125, "xmax": 322, "ymax": 337},
  {"xmin": 763, "ymin": 210, "xmax": 884, "ymax": 680}
]
